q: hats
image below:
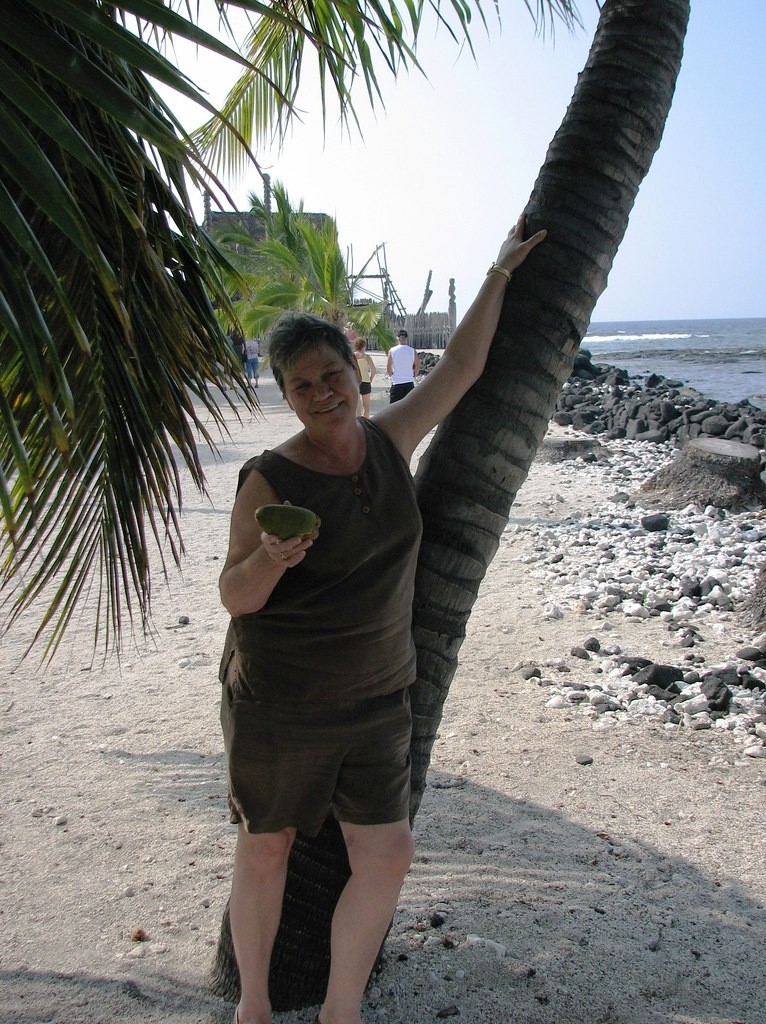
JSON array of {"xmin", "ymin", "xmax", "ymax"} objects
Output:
[{"xmin": 398, "ymin": 330, "xmax": 408, "ymax": 338}]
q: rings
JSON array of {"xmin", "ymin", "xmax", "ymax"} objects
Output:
[{"xmin": 279, "ymin": 552, "xmax": 287, "ymax": 559}]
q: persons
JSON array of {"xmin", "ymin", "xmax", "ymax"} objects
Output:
[
  {"xmin": 216, "ymin": 335, "xmax": 237, "ymax": 391},
  {"xmin": 387, "ymin": 330, "xmax": 420, "ymax": 404},
  {"xmin": 352, "ymin": 337, "xmax": 376, "ymax": 418},
  {"xmin": 242, "ymin": 341, "xmax": 259, "ymax": 389},
  {"xmin": 216, "ymin": 224, "xmax": 544, "ymax": 1024}
]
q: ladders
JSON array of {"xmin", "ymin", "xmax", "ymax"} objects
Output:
[{"xmin": 382, "ymin": 267, "xmax": 407, "ymax": 317}]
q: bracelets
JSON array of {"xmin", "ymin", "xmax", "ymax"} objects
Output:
[{"xmin": 486, "ymin": 262, "xmax": 512, "ymax": 281}]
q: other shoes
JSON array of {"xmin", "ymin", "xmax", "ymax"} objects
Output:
[
  {"xmin": 315, "ymin": 1014, "xmax": 322, "ymax": 1024},
  {"xmin": 234, "ymin": 1003, "xmax": 244, "ymax": 1024},
  {"xmin": 230, "ymin": 387, "xmax": 237, "ymax": 390},
  {"xmin": 255, "ymin": 385, "xmax": 258, "ymax": 388},
  {"xmin": 247, "ymin": 386, "xmax": 252, "ymax": 388},
  {"xmin": 224, "ymin": 388, "xmax": 228, "ymax": 391}
]
